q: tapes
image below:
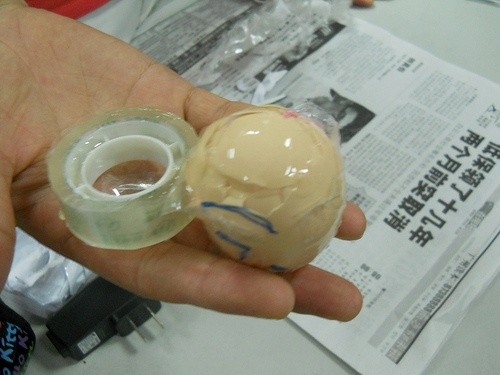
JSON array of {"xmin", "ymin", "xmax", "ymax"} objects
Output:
[{"xmin": 45, "ymin": 103, "xmax": 200, "ymax": 251}]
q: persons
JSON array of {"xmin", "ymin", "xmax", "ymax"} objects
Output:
[{"xmin": 0, "ymin": 0, "xmax": 367, "ymax": 322}]
